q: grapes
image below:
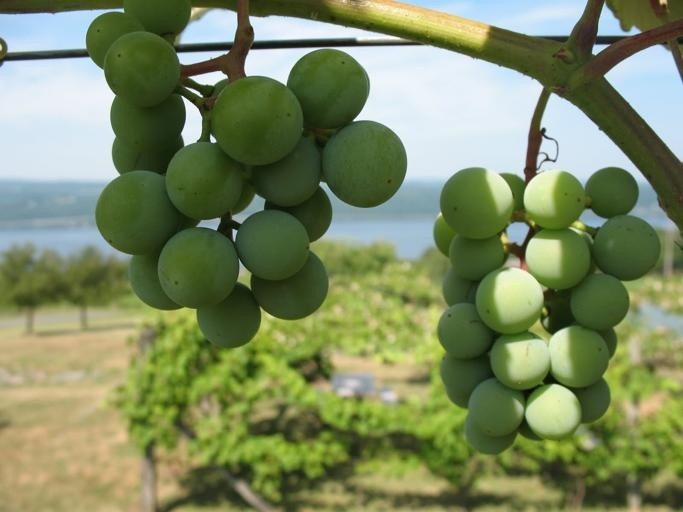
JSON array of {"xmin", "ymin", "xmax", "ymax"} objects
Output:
[
  {"xmin": 412, "ymin": 85, "xmax": 662, "ymax": 456},
  {"xmin": 71, "ymin": 0, "xmax": 407, "ymax": 350}
]
